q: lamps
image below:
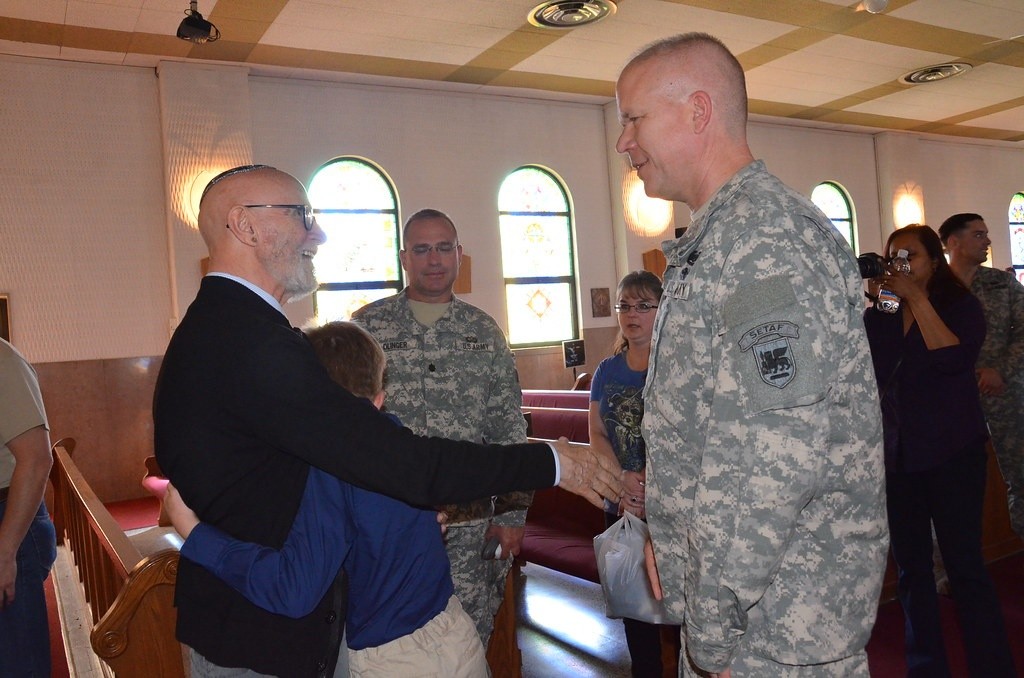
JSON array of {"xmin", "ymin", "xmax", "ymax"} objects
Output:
[{"xmin": 177, "ymin": 0, "xmax": 221, "ymax": 43}]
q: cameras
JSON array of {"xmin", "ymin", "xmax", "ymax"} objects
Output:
[{"xmin": 857, "ymin": 252, "xmax": 887, "ymax": 278}]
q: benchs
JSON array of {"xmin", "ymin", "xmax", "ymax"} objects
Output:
[
  {"xmin": 520, "ymin": 406, "xmax": 602, "ymax": 583},
  {"xmin": 47, "ymin": 435, "xmax": 191, "ymax": 678}
]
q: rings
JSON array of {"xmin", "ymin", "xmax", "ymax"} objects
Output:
[{"xmin": 631, "ymin": 496, "xmax": 638, "ymax": 502}]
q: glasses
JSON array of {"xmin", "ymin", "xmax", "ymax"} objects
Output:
[
  {"xmin": 614, "ymin": 304, "xmax": 658, "ymax": 313},
  {"xmin": 412, "ymin": 245, "xmax": 455, "ymax": 256},
  {"xmin": 226, "ymin": 204, "xmax": 315, "ymax": 231}
]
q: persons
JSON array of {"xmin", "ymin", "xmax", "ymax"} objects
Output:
[
  {"xmin": 0, "ymin": 338, "xmax": 57, "ymax": 678},
  {"xmin": 589, "ymin": 269, "xmax": 683, "ymax": 678},
  {"xmin": 863, "ymin": 224, "xmax": 1010, "ymax": 678},
  {"xmin": 938, "ymin": 213, "xmax": 1024, "ymax": 538},
  {"xmin": 1005, "ymin": 267, "xmax": 1016, "ymax": 277},
  {"xmin": 614, "ymin": 33, "xmax": 889, "ymax": 678},
  {"xmin": 142, "ymin": 164, "xmax": 626, "ymax": 677}
]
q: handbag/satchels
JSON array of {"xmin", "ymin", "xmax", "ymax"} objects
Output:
[{"xmin": 592, "ymin": 509, "xmax": 676, "ymax": 626}]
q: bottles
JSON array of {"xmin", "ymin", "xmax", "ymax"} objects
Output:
[{"xmin": 877, "ymin": 250, "xmax": 910, "ymax": 313}]
